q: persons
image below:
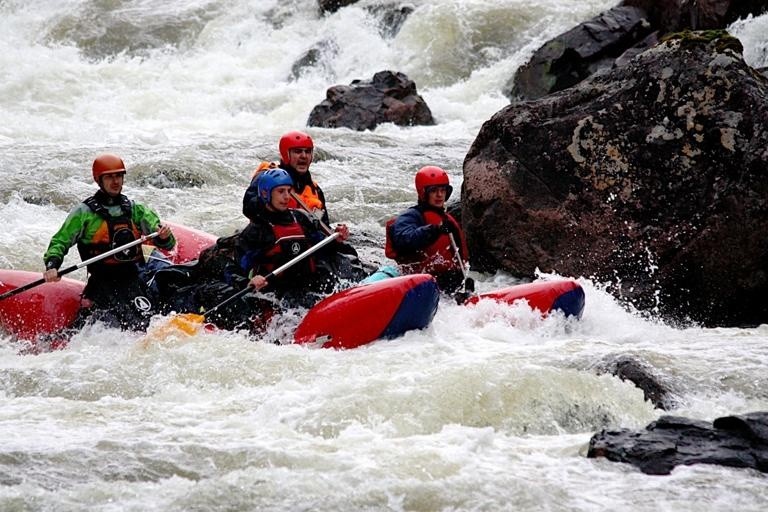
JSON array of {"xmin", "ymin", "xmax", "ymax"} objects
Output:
[
  {"xmin": 242, "ymin": 131, "xmax": 368, "ymax": 284},
  {"xmin": 42, "ymin": 156, "xmax": 176, "ymax": 343},
  {"xmin": 389, "ymin": 167, "xmax": 471, "ymax": 300},
  {"xmin": 223, "ymin": 169, "xmax": 348, "ymax": 322}
]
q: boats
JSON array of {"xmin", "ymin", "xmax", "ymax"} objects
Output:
[
  {"xmin": 154, "ymin": 222, "xmax": 586, "ymax": 334},
  {"xmin": 0, "ymin": 268, "xmax": 442, "ymax": 358}
]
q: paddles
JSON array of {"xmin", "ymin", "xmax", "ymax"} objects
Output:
[{"xmin": 161, "ymin": 224, "xmax": 341, "ymax": 339}]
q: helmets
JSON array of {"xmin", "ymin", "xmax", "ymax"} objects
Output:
[
  {"xmin": 258, "ymin": 168, "xmax": 294, "ymax": 204},
  {"xmin": 93, "ymin": 155, "xmax": 126, "ymax": 181},
  {"xmin": 279, "ymin": 131, "xmax": 313, "ymax": 165},
  {"xmin": 416, "ymin": 166, "xmax": 452, "ymax": 202}
]
